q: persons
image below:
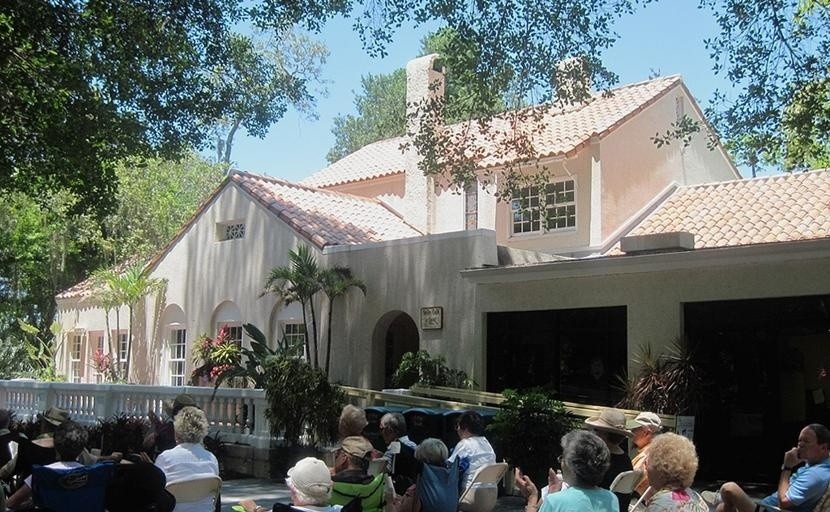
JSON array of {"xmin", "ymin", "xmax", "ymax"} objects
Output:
[
  {"xmin": 701, "ymin": 424, "xmax": 829, "ymax": 512},
  {"xmin": 1, "ymin": 395, "xmax": 222, "ymax": 512},
  {"xmin": 268, "ymin": 404, "xmax": 709, "ymax": 512}
]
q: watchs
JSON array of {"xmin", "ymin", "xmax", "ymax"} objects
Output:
[{"xmin": 781, "ymin": 464, "xmax": 792, "ymax": 471}]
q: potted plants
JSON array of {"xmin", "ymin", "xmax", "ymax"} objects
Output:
[{"xmin": 258, "ymin": 337, "xmax": 348, "ymax": 471}]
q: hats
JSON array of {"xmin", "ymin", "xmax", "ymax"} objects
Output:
[
  {"xmin": 329, "ymin": 435, "xmax": 375, "ymax": 461},
  {"xmin": 36, "ymin": 405, "xmax": 72, "ymax": 427},
  {"xmin": 583, "ymin": 408, "xmax": 636, "ymax": 438},
  {"xmin": 625, "ymin": 410, "xmax": 664, "ymax": 433},
  {"xmin": 286, "ymin": 455, "xmax": 333, "ymax": 498},
  {"xmin": 172, "ymin": 392, "xmax": 202, "ymax": 410}
]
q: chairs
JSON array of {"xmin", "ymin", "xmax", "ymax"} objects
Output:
[
  {"xmin": 0, "ymin": 431, "xmax": 223, "ymax": 512},
  {"xmin": 607, "ymin": 470, "xmax": 830, "ymax": 512},
  {"xmin": 273, "ymin": 437, "xmax": 508, "ymax": 512}
]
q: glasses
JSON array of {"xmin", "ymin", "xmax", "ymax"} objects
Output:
[{"xmin": 557, "ymin": 453, "xmax": 569, "ymax": 464}]
